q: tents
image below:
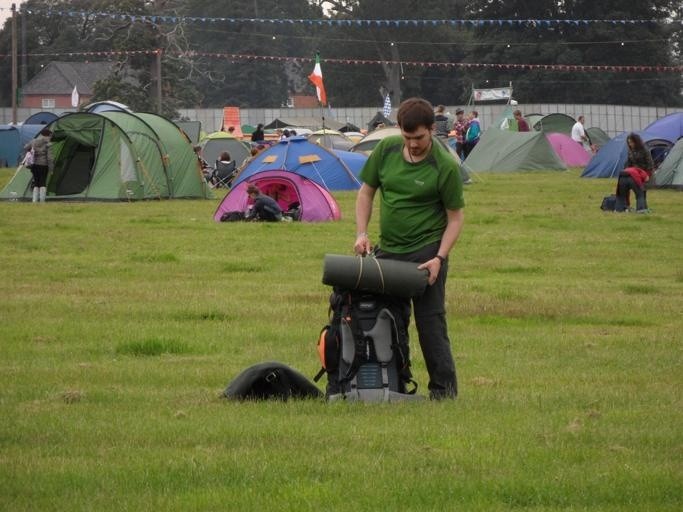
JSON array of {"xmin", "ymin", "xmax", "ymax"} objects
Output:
[
  {"xmin": 1, "ymin": 100, "xmax": 402, "ymax": 219},
  {"xmin": 436, "ymin": 110, "xmax": 682, "ymax": 188}
]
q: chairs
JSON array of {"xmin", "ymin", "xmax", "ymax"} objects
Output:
[{"xmin": 216, "ymin": 159, "xmax": 236, "ymax": 190}]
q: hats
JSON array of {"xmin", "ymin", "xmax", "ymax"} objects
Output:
[{"xmin": 456, "ymin": 108, "xmax": 464, "ymax": 115}]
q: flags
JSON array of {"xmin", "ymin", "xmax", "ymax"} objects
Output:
[{"xmin": 307, "ymin": 55, "xmax": 328, "ymax": 106}]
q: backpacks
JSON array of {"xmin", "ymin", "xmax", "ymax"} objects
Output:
[
  {"xmin": 313, "ymin": 246, "xmax": 426, "ymax": 405},
  {"xmin": 220, "ymin": 211, "xmax": 246, "ymax": 222}
]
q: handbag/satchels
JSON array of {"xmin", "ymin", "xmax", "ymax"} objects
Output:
[
  {"xmin": 22, "ymin": 147, "xmax": 35, "ymax": 168},
  {"xmin": 601, "ymin": 194, "xmax": 616, "ymax": 210},
  {"xmin": 221, "ymin": 361, "xmax": 324, "ymax": 401}
]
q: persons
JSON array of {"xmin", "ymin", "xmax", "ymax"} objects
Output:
[
  {"xmin": 613, "ymin": 133, "xmax": 654, "ymax": 214},
  {"xmin": 433, "ymin": 104, "xmax": 480, "ymax": 158},
  {"xmin": 356, "ymin": 99, "xmax": 465, "ymax": 398}
]
